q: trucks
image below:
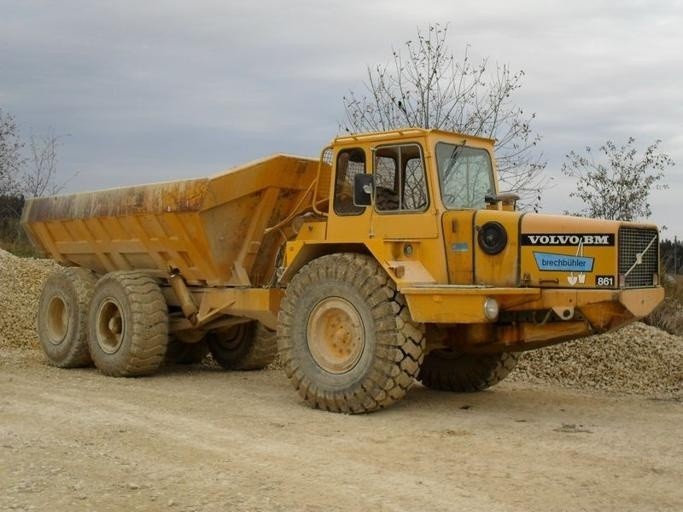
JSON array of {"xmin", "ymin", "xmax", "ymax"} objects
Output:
[{"xmin": 17, "ymin": 122, "xmax": 663, "ymax": 424}]
